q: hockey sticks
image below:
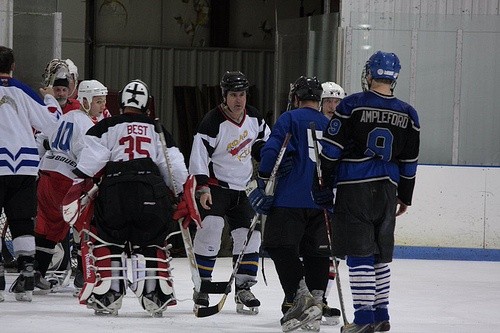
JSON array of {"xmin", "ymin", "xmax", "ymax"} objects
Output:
[
  {"xmin": 194, "ymin": 131, "xmax": 293, "ymax": 318},
  {"xmin": 310, "ymin": 124, "xmax": 350, "ymax": 327},
  {"xmin": 2, "ymin": 72, "xmax": 57, "ymax": 267},
  {"xmin": 155, "ymin": 116, "xmax": 232, "ymax": 296}
]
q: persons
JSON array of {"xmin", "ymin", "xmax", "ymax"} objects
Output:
[
  {"xmin": 312, "ymin": 51, "xmax": 421, "ymax": 333},
  {"xmin": 0, "ymin": 47, "xmax": 342, "ymax": 332}
]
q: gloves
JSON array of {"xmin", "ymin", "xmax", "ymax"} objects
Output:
[
  {"xmin": 311, "ymin": 179, "xmax": 335, "ymax": 209},
  {"xmin": 245, "ymin": 174, "xmax": 276, "ymax": 215}
]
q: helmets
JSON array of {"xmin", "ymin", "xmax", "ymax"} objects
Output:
[
  {"xmin": 77, "ymin": 80, "xmax": 108, "ymax": 112},
  {"xmin": 287, "ymin": 76, "xmax": 323, "ymax": 110},
  {"xmin": 220, "ymin": 71, "xmax": 249, "ymax": 98},
  {"xmin": 366, "ymin": 51, "xmax": 401, "ymax": 81},
  {"xmin": 120, "ymin": 79, "xmax": 152, "ymax": 112},
  {"xmin": 319, "ymin": 81, "xmax": 345, "ymax": 112},
  {"xmin": 65, "ymin": 59, "xmax": 79, "ymax": 97}
]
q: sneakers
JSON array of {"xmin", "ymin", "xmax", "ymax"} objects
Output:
[
  {"xmin": 141, "ymin": 290, "xmax": 168, "ymax": 318},
  {"xmin": 192, "ymin": 287, "xmax": 209, "ymax": 315},
  {"xmin": 311, "ymin": 290, "xmax": 341, "ymax": 326},
  {"xmin": 9, "ymin": 269, "xmax": 34, "ymax": 302},
  {"xmin": 92, "ymin": 288, "xmax": 122, "ymax": 316},
  {"xmin": 0, "ymin": 270, "xmax": 6, "ymax": 302},
  {"xmin": 280, "ymin": 277, "xmax": 322, "ymax": 333},
  {"xmin": 234, "ymin": 284, "xmax": 261, "ymax": 315},
  {"xmin": 33, "ymin": 271, "xmax": 51, "ymax": 295},
  {"xmin": 281, "ymin": 295, "xmax": 321, "ymax": 331},
  {"xmin": 340, "ymin": 319, "xmax": 391, "ymax": 333}
]
